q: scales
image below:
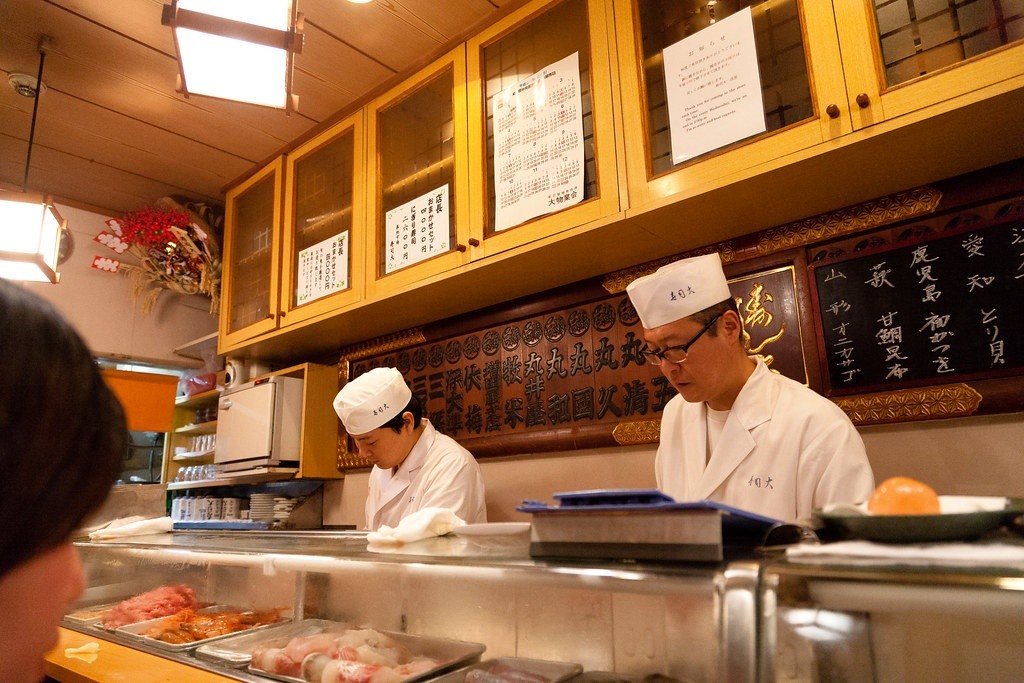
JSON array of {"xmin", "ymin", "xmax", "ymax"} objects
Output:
[{"xmin": 516, "ymin": 488, "xmax": 803, "ymax": 566}]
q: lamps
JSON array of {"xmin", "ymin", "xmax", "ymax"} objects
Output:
[{"xmin": 1, "ymin": 35, "xmax": 72, "ymax": 281}]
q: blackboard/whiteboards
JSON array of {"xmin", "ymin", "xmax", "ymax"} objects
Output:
[{"xmin": 806, "ymin": 210, "xmax": 1024, "ymax": 398}]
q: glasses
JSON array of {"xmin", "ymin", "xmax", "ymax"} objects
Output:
[{"xmin": 639, "ymin": 312, "xmax": 724, "ymax": 366}]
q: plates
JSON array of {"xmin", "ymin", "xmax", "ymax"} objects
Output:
[
  {"xmin": 815, "ymin": 495, "xmax": 1024, "ymax": 542},
  {"xmin": 248, "ymin": 493, "xmax": 305, "ymax": 522}
]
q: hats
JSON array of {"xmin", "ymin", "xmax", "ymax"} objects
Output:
[
  {"xmin": 332, "ymin": 367, "xmax": 412, "ymax": 435},
  {"xmin": 625, "ymin": 252, "xmax": 732, "ymax": 330}
]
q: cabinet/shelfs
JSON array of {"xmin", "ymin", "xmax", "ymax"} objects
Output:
[
  {"xmin": 607, "ymin": 1, "xmax": 1023, "ymax": 221},
  {"xmin": 41, "ymin": 519, "xmax": 1024, "ymax": 683},
  {"xmin": 364, "ymin": 0, "xmax": 629, "ymax": 303},
  {"xmin": 218, "ymin": 106, "xmax": 365, "ymax": 358},
  {"xmin": 166, "ymin": 362, "xmax": 346, "ymax": 481}
]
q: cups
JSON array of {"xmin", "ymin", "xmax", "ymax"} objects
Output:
[
  {"xmin": 189, "ymin": 434, "xmax": 216, "ymax": 452},
  {"xmin": 175, "ymin": 464, "xmax": 218, "ymax": 481}
]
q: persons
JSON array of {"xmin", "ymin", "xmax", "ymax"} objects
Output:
[
  {"xmin": 625, "ymin": 251, "xmax": 875, "ymax": 546},
  {"xmin": 0, "ymin": 279, "xmax": 136, "ymax": 683},
  {"xmin": 331, "ymin": 365, "xmax": 489, "ymax": 537}
]
